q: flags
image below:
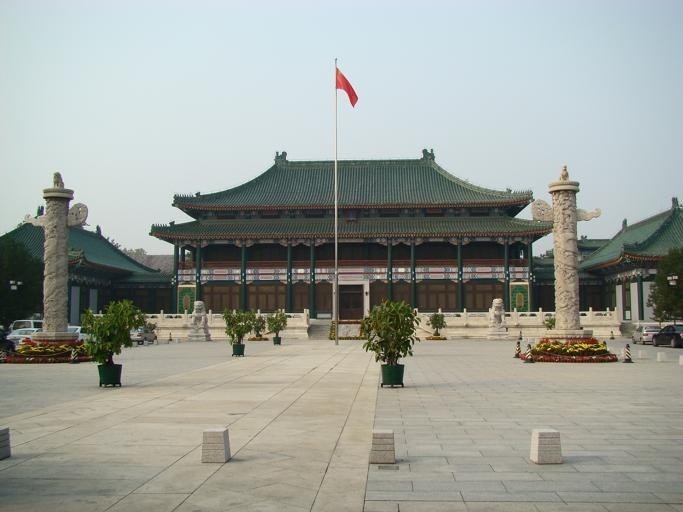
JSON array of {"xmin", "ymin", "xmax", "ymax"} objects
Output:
[{"xmin": 336, "ymin": 66, "xmax": 359, "ymax": 108}]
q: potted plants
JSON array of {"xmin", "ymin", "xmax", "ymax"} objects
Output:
[
  {"xmin": 79, "ymin": 298, "xmax": 150, "ymax": 389},
  {"xmin": 428, "ymin": 312, "xmax": 446, "ymax": 337},
  {"xmin": 223, "ymin": 306, "xmax": 291, "ymax": 357},
  {"xmin": 359, "ymin": 297, "xmax": 421, "ymax": 387}
]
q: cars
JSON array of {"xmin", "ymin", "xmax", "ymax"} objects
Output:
[
  {"xmin": 630, "ymin": 324, "xmax": 682, "ymax": 348},
  {"xmin": 0, "ymin": 319, "xmax": 97, "ymax": 352},
  {"xmin": 129, "ymin": 327, "xmax": 157, "ymax": 345}
]
